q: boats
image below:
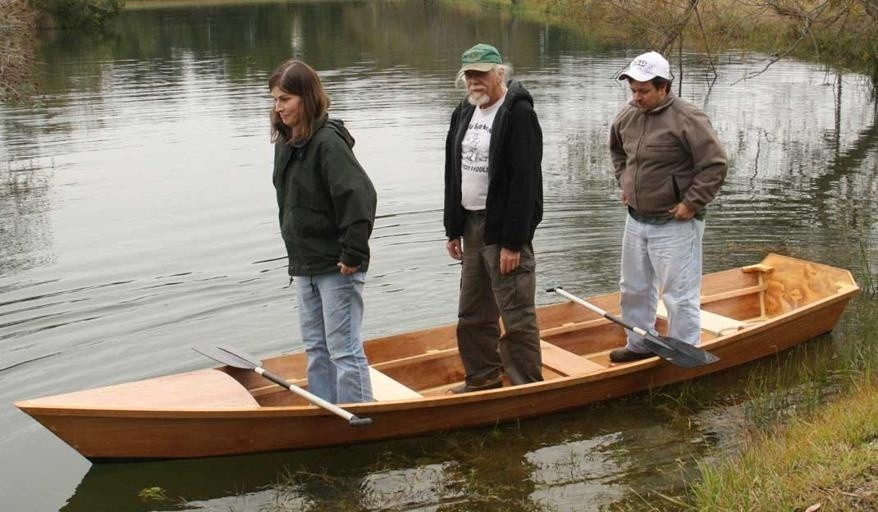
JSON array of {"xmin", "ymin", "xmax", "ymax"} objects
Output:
[{"xmin": 12, "ymin": 252, "xmax": 860, "ymax": 458}]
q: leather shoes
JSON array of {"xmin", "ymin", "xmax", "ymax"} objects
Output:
[
  {"xmin": 608, "ymin": 346, "xmax": 655, "ymax": 362},
  {"xmin": 447, "ymin": 377, "xmax": 503, "ymax": 395}
]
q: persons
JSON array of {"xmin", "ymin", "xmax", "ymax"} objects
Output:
[
  {"xmin": 442, "ymin": 42, "xmax": 544, "ymax": 398},
  {"xmin": 609, "ymin": 49, "xmax": 728, "ymax": 365},
  {"xmin": 269, "ymin": 60, "xmax": 377, "ymax": 409}
]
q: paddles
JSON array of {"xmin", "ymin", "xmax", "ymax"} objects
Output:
[
  {"xmin": 193, "ymin": 344, "xmax": 372, "ymax": 427},
  {"xmin": 547, "ymin": 286, "xmax": 721, "ymax": 369}
]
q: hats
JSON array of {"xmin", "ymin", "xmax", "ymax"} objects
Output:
[
  {"xmin": 457, "ymin": 42, "xmax": 502, "ymax": 71},
  {"xmin": 618, "ymin": 50, "xmax": 674, "ymax": 83}
]
